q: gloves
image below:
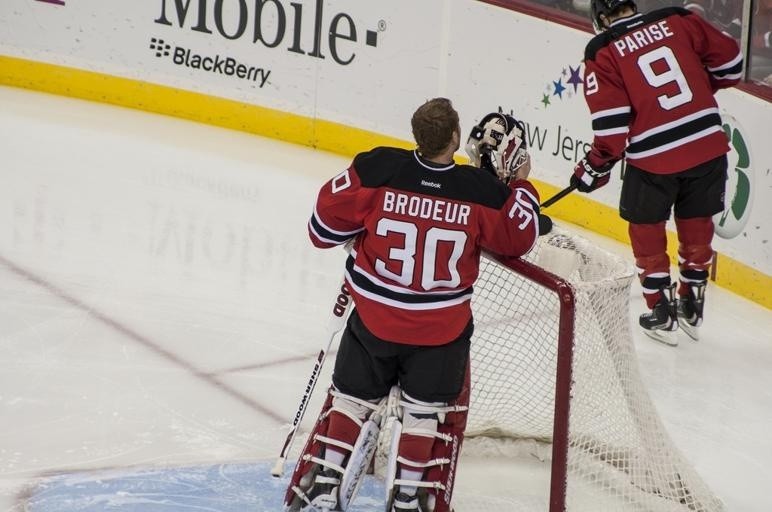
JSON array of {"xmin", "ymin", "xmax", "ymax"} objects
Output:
[{"xmin": 569, "ymin": 151, "xmax": 611, "ymax": 194}]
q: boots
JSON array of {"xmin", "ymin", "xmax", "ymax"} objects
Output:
[
  {"xmin": 392, "ymin": 493, "xmax": 420, "ymax": 512},
  {"xmin": 679, "ymin": 278, "xmax": 709, "ymax": 329},
  {"xmin": 638, "ymin": 281, "xmax": 679, "ymax": 335},
  {"xmin": 295, "ymin": 468, "xmax": 338, "ymax": 512}
]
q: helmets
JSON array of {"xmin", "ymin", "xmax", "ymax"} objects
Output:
[
  {"xmin": 462, "ymin": 111, "xmax": 532, "ymax": 185},
  {"xmin": 589, "ymin": 0, "xmax": 636, "ymax": 36}
]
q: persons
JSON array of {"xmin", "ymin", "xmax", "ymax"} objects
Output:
[
  {"xmin": 571, "ymin": 2, "xmax": 738, "ymax": 345},
  {"xmin": 275, "ymin": 102, "xmax": 541, "ymax": 511}
]
